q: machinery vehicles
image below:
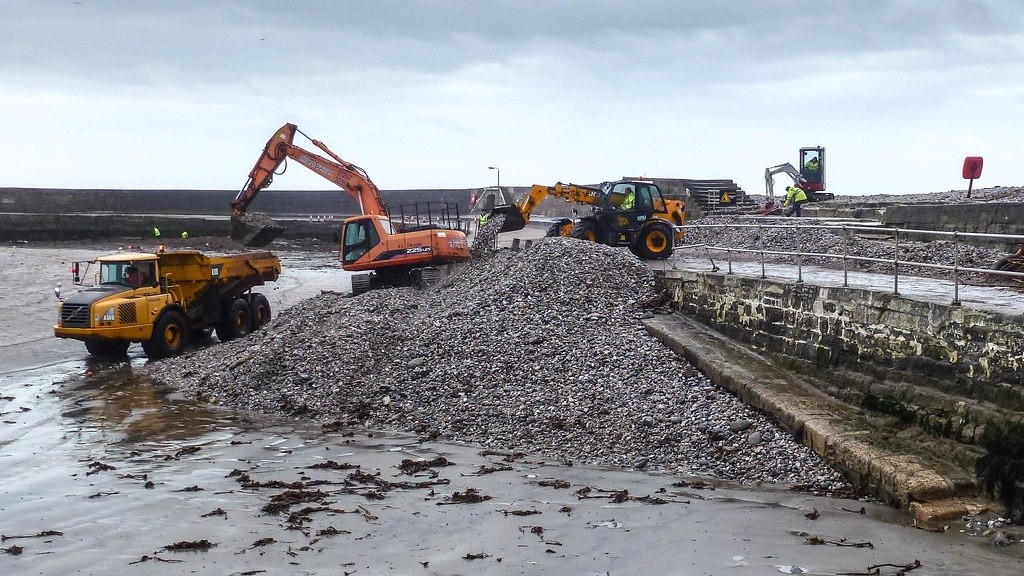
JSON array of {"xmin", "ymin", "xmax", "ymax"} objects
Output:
[
  {"xmin": 227, "ymin": 124, "xmax": 474, "ymax": 297},
  {"xmin": 488, "ymin": 178, "xmax": 689, "ymax": 262},
  {"xmin": 763, "ymin": 144, "xmax": 837, "ymax": 210}
]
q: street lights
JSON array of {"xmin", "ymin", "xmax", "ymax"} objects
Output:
[{"xmin": 489, "ymin": 166, "xmax": 500, "ymax": 207}]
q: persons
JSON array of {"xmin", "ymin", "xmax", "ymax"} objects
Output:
[
  {"xmin": 805, "ymin": 157, "xmax": 820, "ymax": 171},
  {"xmin": 616, "ymin": 188, "xmax": 636, "ymax": 212},
  {"xmin": 181, "ymin": 230, "xmax": 189, "ymax": 240},
  {"xmin": 124, "ymin": 267, "xmax": 147, "ymax": 287},
  {"xmin": 782, "ymin": 185, "xmax": 807, "ymax": 222},
  {"xmin": 478, "ymin": 209, "xmax": 490, "ymax": 233},
  {"xmin": 153, "ymin": 226, "xmax": 162, "ymax": 241}
]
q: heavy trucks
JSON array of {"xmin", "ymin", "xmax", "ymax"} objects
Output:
[{"xmin": 51, "ymin": 245, "xmax": 284, "ymax": 359}]
